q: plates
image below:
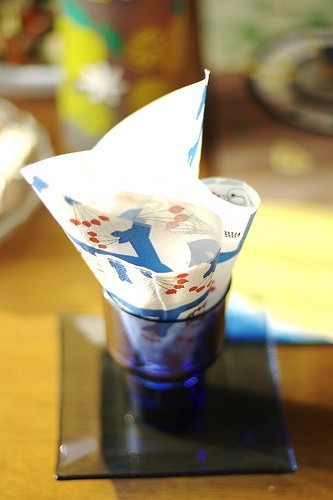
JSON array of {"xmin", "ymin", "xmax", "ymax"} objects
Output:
[
  {"xmin": 1, "ymin": 100, "xmax": 55, "ymax": 241},
  {"xmin": 250, "ymin": 26, "xmax": 333, "ymax": 137},
  {"xmin": 54, "ymin": 312, "xmax": 299, "ymax": 480}
]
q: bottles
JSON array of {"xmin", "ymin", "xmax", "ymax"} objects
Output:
[{"xmin": 50, "ymin": 0, "xmax": 198, "ymax": 155}]
